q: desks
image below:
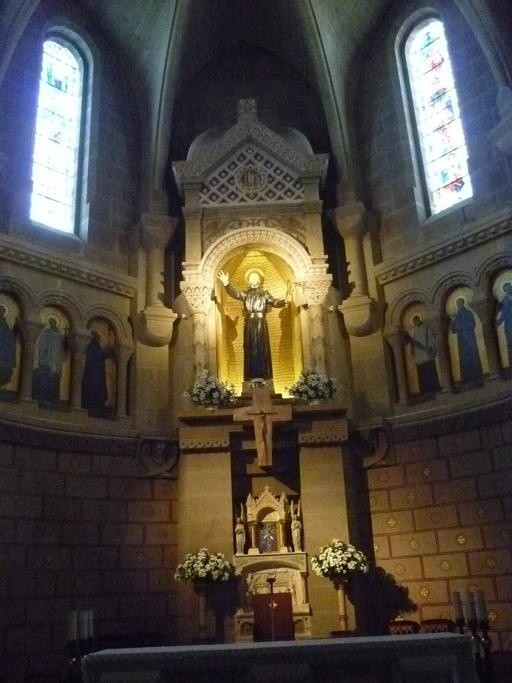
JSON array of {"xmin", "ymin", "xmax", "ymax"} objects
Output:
[{"xmin": 83, "ymin": 629, "xmax": 473, "ymax": 683}]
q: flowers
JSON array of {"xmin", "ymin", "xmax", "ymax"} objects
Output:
[
  {"xmin": 173, "ymin": 545, "xmax": 230, "ymax": 582},
  {"xmin": 288, "ymin": 366, "xmax": 338, "ymax": 398},
  {"xmin": 309, "ymin": 537, "xmax": 371, "ymax": 581},
  {"xmin": 182, "ymin": 369, "xmax": 234, "ymax": 405}
]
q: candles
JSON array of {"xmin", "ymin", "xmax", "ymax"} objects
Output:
[
  {"xmin": 452, "ymin": 589, "xmax": 487, "ymax": 621},
  {"xmin": 65, "ymin": 607, "xmax": 94, "ymax": 639}
]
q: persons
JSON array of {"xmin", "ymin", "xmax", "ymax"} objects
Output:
[
  {"xmin": 291, "ymin": 514, "xmax": 303, "ymax": 552},
  {"xmin": 410, "ymin": 282, "xmax": 512, "ymax": 395},
  {"xmin": 0, "ymin": 305, "xmax": 113, "ymax": 420},
  {"xmin": 217, "ymin": 269, "xmax": 289, "ymax": 384},
  {"xmin": 235, "ymin": 517, "xmax": 246, "ymax": 555}
]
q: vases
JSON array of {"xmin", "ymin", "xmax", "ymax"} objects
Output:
[
  {"xmin": 190, "ymin": 578, "xmax": 216, "ymax": 639},
  {"xmin": 330, "ymin": 572, "xmax": 352, "ymax": 636}
]
